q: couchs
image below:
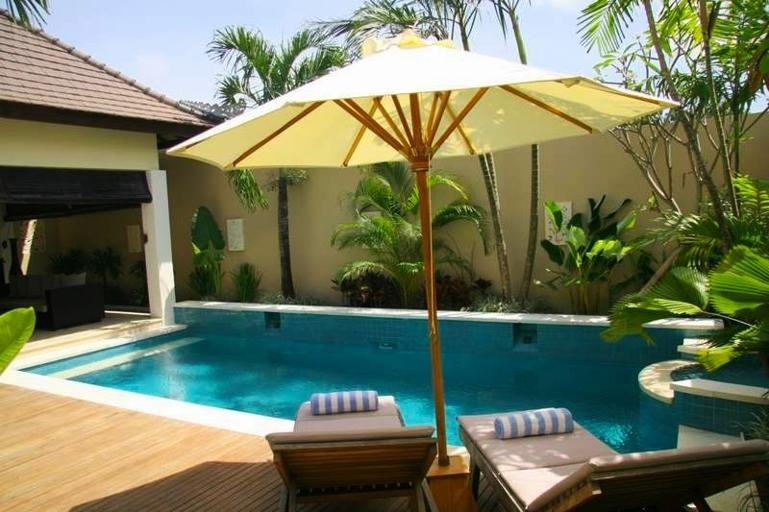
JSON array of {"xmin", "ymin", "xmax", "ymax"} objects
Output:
[{"xmin": 0, "ymin": 282, "xmax": 105, "ymax": 331}]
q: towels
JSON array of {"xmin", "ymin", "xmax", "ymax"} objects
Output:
[
  {"xmin": 495, "ymin": 407, "xmax": 573, "ymax": 438},
  {"xmin": 311, "ymin": 391, "xmax": 378, "ymax": 414}
]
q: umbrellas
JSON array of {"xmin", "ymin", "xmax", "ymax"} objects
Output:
[{"xmin": 163, "ymin": 26, "xmax": 682, "ymax": 466}]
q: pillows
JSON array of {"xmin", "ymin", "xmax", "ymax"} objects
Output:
[{"xmin": 10, "ymin": 272, "xmax": 86, "ymax": 298}]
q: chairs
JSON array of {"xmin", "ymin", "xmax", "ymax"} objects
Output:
[
  {"xmin": 266, "ymin": 394, "xmax": 438, "ymax": 511},
  {"xmin": 456, "ymin": 407, "xmax": 769, "ymax": 512}
]
